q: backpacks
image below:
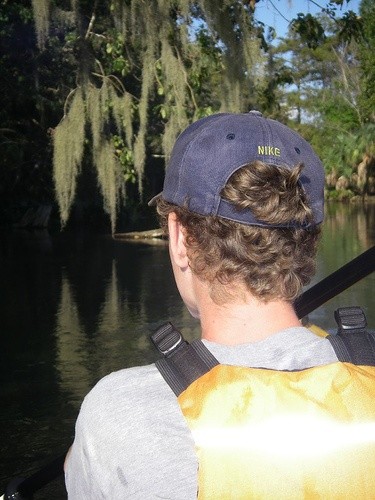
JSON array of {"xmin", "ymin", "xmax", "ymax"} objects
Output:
[{"xmin": 150, "ymin": 307, "xmax": 374, "ymax": 500}]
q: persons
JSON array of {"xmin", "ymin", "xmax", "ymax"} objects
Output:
[{"xmin": 63, "ymin": 109, "xmax": 375, "ymax": 499}]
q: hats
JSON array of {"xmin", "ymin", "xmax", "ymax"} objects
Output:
[{"xmin": 148, "ymin": 112, "xmax": 324, "ymax": 228}]
